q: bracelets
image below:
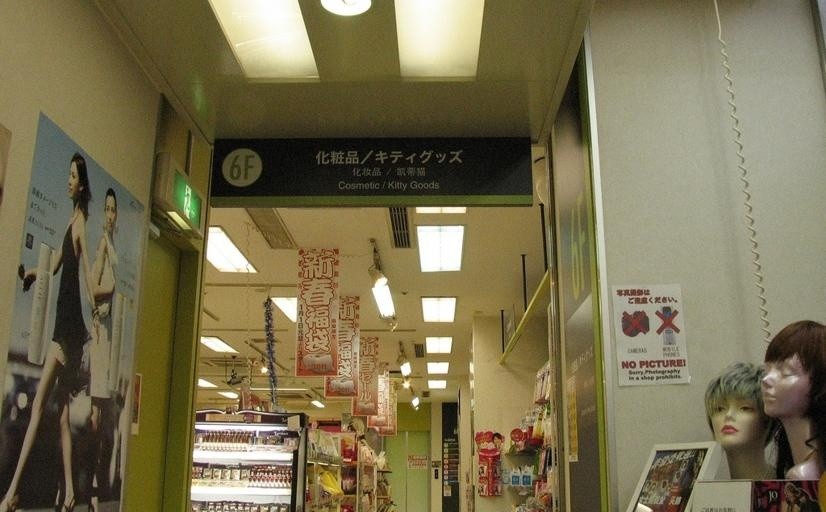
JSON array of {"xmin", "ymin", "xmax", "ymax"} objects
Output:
[{"xmin": 91, "ymin": 309, "xmax": 99, "ymax": 319}]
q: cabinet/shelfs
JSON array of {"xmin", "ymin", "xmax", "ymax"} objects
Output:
[
  {"xmin": 499, "ymin": 360, "xmax": 561, "ymax": 512},
  {"xmin": 189, "ymin": 423, "xmax": 294, "ymax": 512},
  {"xmin": 305, "ymin": 432, "xmax": 398, "ymax": 512}
]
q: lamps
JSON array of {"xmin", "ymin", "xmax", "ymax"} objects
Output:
[
  {"xmin": 321, "ymin": 0, "xmax": 373, "ymax": 17},
  {"xmin": 367, "ymin": 266, "xmax": 420, "ymax": 412}
]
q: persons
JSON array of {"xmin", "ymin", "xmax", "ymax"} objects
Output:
[
  {"xmin": 760, "ymin": 319, "xmax": 826, "ymax": 481},
  {"xmin": 492, "ymin": 432, "xmax": 503, "ymax": 449},
  {"xmin": 702, "ymin": 360, "xmax": 780, "ymax": 480},
  {"xmin": 0, "ymin": 152, "xmax": 101, "ymax": 512},
  {"xmin": 78, "ymin": 188, "xmax": 119, "ymax": 503}
]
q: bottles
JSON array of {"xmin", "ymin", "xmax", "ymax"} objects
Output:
[
  {"xmin": 203, "ymin": 429, "xmax": 252, "ymax": 444},
  {"xmin": 248, "ymin": 466, "xmax": 292, "ymax": 487}
]
